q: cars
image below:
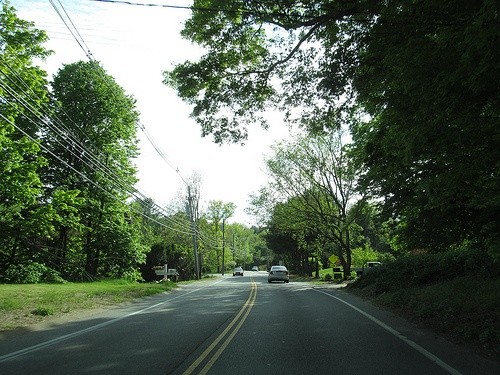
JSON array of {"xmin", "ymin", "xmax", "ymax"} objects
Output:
[
  {"xmin": 233, "ymin": 267, "xmax": 243, "ymax": 276},
  {"xmin": 267, "ymin": 265, "xmax": 290, "ymax": 283},
  {"xmin": 252, "ymin": 266, "xmax": 258, "ymax": 271}
]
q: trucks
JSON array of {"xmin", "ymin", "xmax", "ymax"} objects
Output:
[{"xmin": 350, "ymin": 262, "xmax": 381, "ymax": 277}]
